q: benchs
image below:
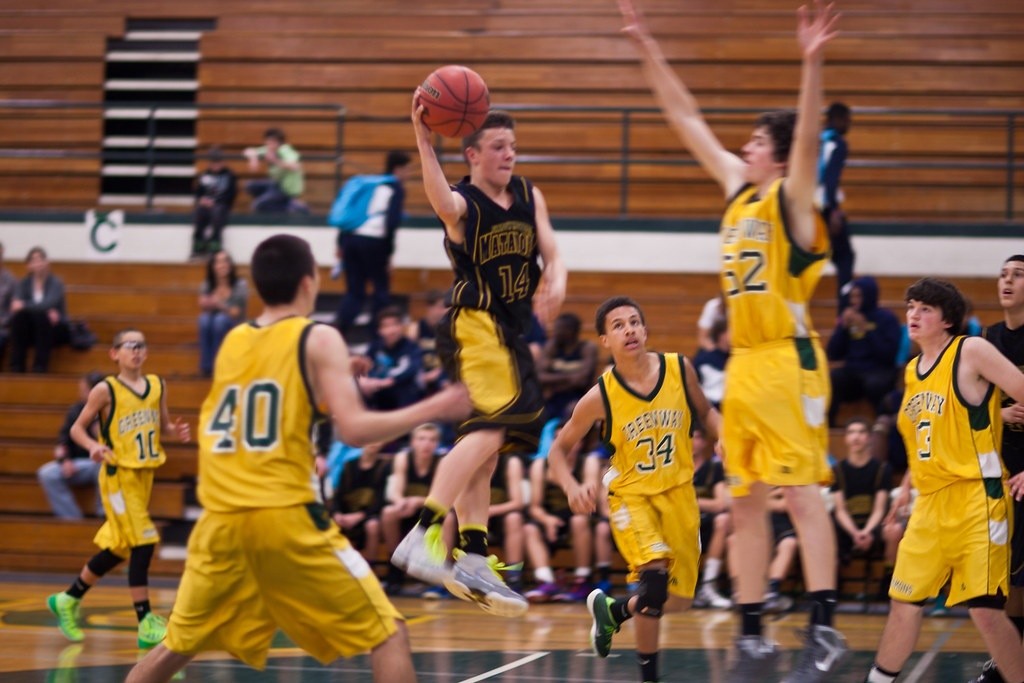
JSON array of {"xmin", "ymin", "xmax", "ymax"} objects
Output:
[{"xmin": 4, "ymin": 0, "xmax": 1024, "ymax": 577}]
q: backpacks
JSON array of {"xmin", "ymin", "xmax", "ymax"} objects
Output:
[{"xmin": 328, "ymin": 174, "xmax": 399, "ymax": 232}]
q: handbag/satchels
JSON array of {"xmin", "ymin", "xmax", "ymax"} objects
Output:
[{"xmin": 68, "ymin": 321, "xmax": 97, "ymax": 348}]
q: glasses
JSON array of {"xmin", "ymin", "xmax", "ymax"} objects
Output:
[{"xmin": 114, "ymin": 341, "xmax": 146, "ymax": 350}]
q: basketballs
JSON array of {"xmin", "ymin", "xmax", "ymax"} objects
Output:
[{"xmin": 418, "ymin": 63, "xmax": 492, "ymax": 139}]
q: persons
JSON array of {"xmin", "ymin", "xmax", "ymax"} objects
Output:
[
  {"xmin": 46, "ymin": 328, "xmax": 191, "ymax": 648},
  {"xmin": 328, "ymin": 148, "xmax": 411, "ymax": 335},
  {"xmin": 813, "ymin": 103, "xmax": 855, "ymax": 325},
  {"xmin": 872, "ymin": 464, "xmax": 951, "ymax": 615},
  {"xmin": 0, "ymin": 241, "xmax": 67, "ymax": 373},
  {"xmin": 970, "ymin": 251, "xmax": 1024, "ymax": 683},
  {"xmin": 900, "ymin": 296, "xmax": 983, "ymax": 410},
  {"xmin": 617, "ymin": 0, "xmax": 847, "ymax": 683},
  {"xmin": 190, "ymin": 151, "xmax": 237, "ymax": 259},
  {"xmin": 123, "ymin": 234, "xmax": 473, "ymax": 683},
  {"xmin": 306, "ymin": 292, "xmax": 839, "ymax": 609},
  {"xmin": 242, "ymin": 128, "xmax": 305, "ymax": 217},
  {"xmin": 196, "ymin": 248, "xmax": 247, "ymax": 378},
  {"xmin": 547, "ymin": 296, "xmax": 724, "ymax": 683},
  {"xmin": 829, "ymin": 423, "xmax": 903, "ymax": 601},
  {"xmin": 390, "ymin": 85, "xmax": 568, "ymax": 619},
  {"xmin": 826, "ymin": 274, "xmax": 899, "ymax": 427},
  {"xmin": 862, "ymin": 275, "xmax": 1024, "ymax": 683}
]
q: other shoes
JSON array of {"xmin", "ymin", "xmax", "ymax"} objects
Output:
[
  {"xmin": 423, "ymin": 587, "xmax": 445, "ymax": 598},
  {"xmin": 967, "ymin": 658, "xmax": 1006, "ymax": 683}
]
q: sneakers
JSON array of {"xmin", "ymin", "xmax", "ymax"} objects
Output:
[
  {"xmin": 782, "ymin": 625, "xmax": 852, "ymax": 683},
  {"xmin": 135, "ymin": 610, "xmax": 169, "ymax": 649},
  {"xmin": 585, "ymin": 588, "xmax": 617, "ymax": 659},
  {"xmin": 444, "ymin": 547, "xmax": 529, "ymax": 618},
  {"xmin": 728, "ymin": 637, "xmax": 773, "ymax": 683},
  {"xmin": 694, "ymin": 580, "xmax": 731, "ymax": 607},
  {"xmin": 525, "ymin": 578, "xmax": 561, "ymax": 600},
  {"xmin": 932, "ymin": 594, "xmax": 950, "ymax": 616},
  {"xmin": 46, "ymin": 591, "xmax": 85, "ymax": 641},
  {"xmin": 45, "ymin": 644, "xmax": 84, "ymax": 683},
  {"xmin": 390, "ymin": 528, "xmax": 451, "ymax": 586},
  {"xmin": 552, "ymin": 582, "xmax": 589, "ymax": 601}
]
